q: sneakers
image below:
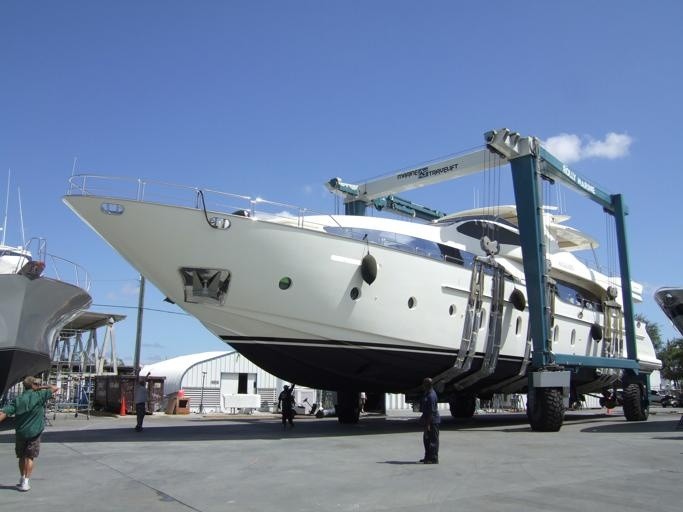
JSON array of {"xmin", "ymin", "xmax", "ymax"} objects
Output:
[{"xmin": 16, "ymin": 483, "xmax": 31, "ymax": 490}]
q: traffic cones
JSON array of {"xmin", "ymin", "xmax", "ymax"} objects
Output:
[{"xmin": 119, "ymin": 395, "xmax": 125, "ymax": 416}]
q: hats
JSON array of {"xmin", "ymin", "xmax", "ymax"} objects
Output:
[{"xmin": 23, "ymin": 377, "xmax": 40, "ymax": 388}]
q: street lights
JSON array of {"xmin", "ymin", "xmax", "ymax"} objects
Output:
[{"xmin": 197, "ymin": 370, "xmax": 208, "ymax": 414}]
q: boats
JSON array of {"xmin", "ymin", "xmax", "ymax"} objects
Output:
[
  {"xmin": 0, "ymin": 162, "xmax": 99, "ymax": 407},
  {"xmin": 56, "ymin": 160, "xmax": 662, "ymax": 398},
  {"xmin": 652, "ymin": 286, "xmax": 683, "ymax": 336}
]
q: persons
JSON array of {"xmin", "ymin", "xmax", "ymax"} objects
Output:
[
  {"xmin": 0, "ymin": 376, "xmax": 59, "ymax": 492},
  {"xmin": 419, "ymin": 377, "xmax": 440, "ymax": 464},
  {"xmin": 133, "ymin": 378, "xmax": 148, "ymax": 432},
  {"xmin": 278, "ymin": 381, "xmax": 296, "ymax": 427}
]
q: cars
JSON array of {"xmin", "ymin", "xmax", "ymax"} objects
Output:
[
  {"xmin": 659, "ymin": 394, "xmax": 680, "ymax": 407},
  {"xmin": 650, "ymin": 388, "xmax": 666, "ymax": 402}
]
